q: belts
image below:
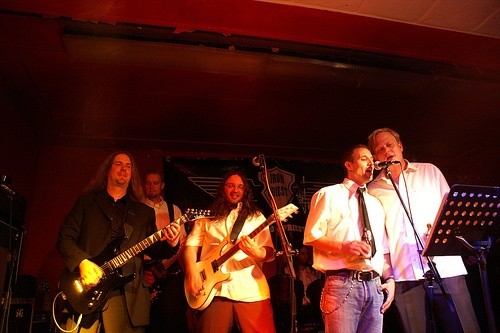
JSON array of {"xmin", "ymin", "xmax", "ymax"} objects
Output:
[{"xmin": 326, "ymin": 269, "xmax": 379, "ymax": 281}]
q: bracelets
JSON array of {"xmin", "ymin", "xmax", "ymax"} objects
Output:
[{"xmin": 385, "ymin": 276, "xmax": 395, "ymax": 283}]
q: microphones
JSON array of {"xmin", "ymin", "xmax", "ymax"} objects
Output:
[
  {"xmin": 374, "ymin": 161, "xmax": 400, "ymax": 170},
  {"xmin": 252, "ymin": 154, "xmax": 264, "ymax": 166}
]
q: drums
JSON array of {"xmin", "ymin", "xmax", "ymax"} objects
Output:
[
  {"xmin": 267, "ymin": 273, "xmax": 304, "ymax": 306},
  {"xmin": 298, "ymin": 316, "xmax": 323, "ymax": 333},
  {"xmin": 306, "ymin": 278, "xmax": 325, "ymax": 319}
]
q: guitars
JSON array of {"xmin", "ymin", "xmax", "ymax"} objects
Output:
[
  {"xmin": 183, "ymin": 203, "xmax": 299, "ymax": 310},
  {"xmin": 143, "ymin": 236, "xmax": 187, "ymax": 287},
  {"xmin": 56, "ymin": 207, "xmax": 217, "ymax": 315}
]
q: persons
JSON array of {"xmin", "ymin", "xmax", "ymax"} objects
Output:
[
  {"xmin": 185, "ymin": 169, "xmax": 276, "ymax": 333},
  {"xmin": 367, "ymin": 127, "xmax": 480, "ymax": 333},
  {"xmin": 54, "ymin": 151, "xmax": 181, "ymax": 333},
  {"xmin": 285, "ymin": 246, "xmax": 319, "ymax": 304},
  {"xmin": 302, "ymin": 144, "xmax": 395, "ymax": 333},
  {"xmin": 140, "ymin": 169, "xmax": 187, "ymax": 333}
]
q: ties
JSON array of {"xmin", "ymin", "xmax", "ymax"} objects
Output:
[{"xmin": 357, "ymin": 187, "xmax": 376, "ymax": 258}]
